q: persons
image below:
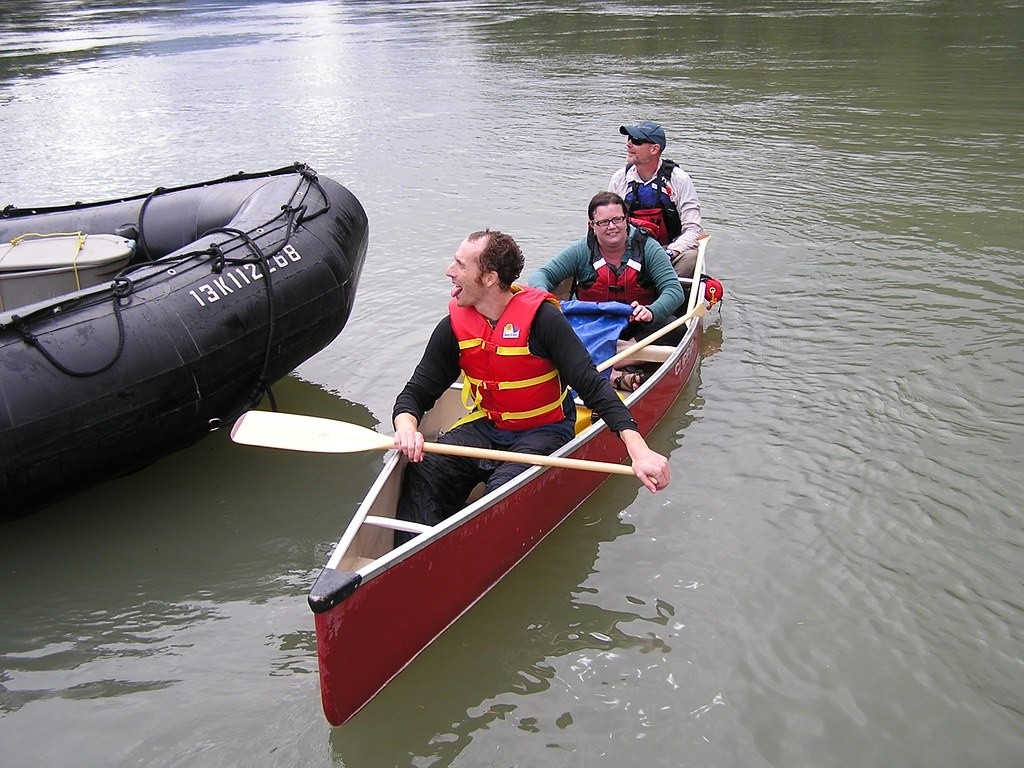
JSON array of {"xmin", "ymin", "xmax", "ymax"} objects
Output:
[
  {"xmin": 393, "ymin": 229, "xmax": 670, "ymax": 550},
  {"xmin": 608, "ymin": 122, "xmax": 701, "ymax": 278},
  {"xmin": 530, "ymin": 193, "xmax": 687, "ymax": 392}
]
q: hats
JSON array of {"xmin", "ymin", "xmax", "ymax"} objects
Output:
[{"xmin": 619, "ymin": 121, "xmax": 666, "ymax": 152}]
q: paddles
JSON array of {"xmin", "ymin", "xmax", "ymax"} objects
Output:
[
  {"xmin": 232, "ymin": 408, "xmax": 659, "ymax": 482},
  {"xmin": 596, "ymin": 301, "xmax": 710, "ymax": 372}
]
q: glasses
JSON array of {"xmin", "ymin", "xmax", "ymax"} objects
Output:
[
  {"xmin": 628, "ymin": 134, "xmax": 656, "ymax": 146},
  {"xmin": 592, "ymin": 213, "xmax": 626, "ymax": 227}
]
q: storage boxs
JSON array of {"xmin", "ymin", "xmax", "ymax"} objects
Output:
[{"xmin": 0, "ymin": 234, "xmax": 136, "ymax": 312}]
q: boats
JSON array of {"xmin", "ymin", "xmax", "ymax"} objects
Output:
[
  {"xmin": 0, "ymin": 162, "xmax": 368, "ymax": 524},
  {"xmin": 308, "ymin": 257, "xmax": 707, "ymax": 728}
]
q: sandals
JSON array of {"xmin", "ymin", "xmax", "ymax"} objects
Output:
[{"xmin": 612, "ymin": 366, "xmax": 645, "ymax": 392}]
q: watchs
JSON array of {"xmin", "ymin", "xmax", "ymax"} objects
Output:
[{"xmin": 666, "ymin": 250, "xmax": 674, "ymax": 259}]
